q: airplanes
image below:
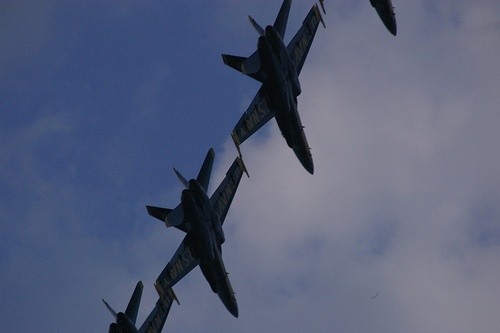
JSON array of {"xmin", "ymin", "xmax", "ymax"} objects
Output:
[
  {"xmin": 318, "ymin": 0, "xmax": 398, "ymax": 38},
  {"xmin": 145, "ymin": 147, "xmax": 250, "ymax": 319},
  {"xmin": 220, "ymin": 0, "xmax": 326, "ymax": 177},
  {"xmin": 101, "ymin": 280, "xmax": 181, "ymax": 332}
]
